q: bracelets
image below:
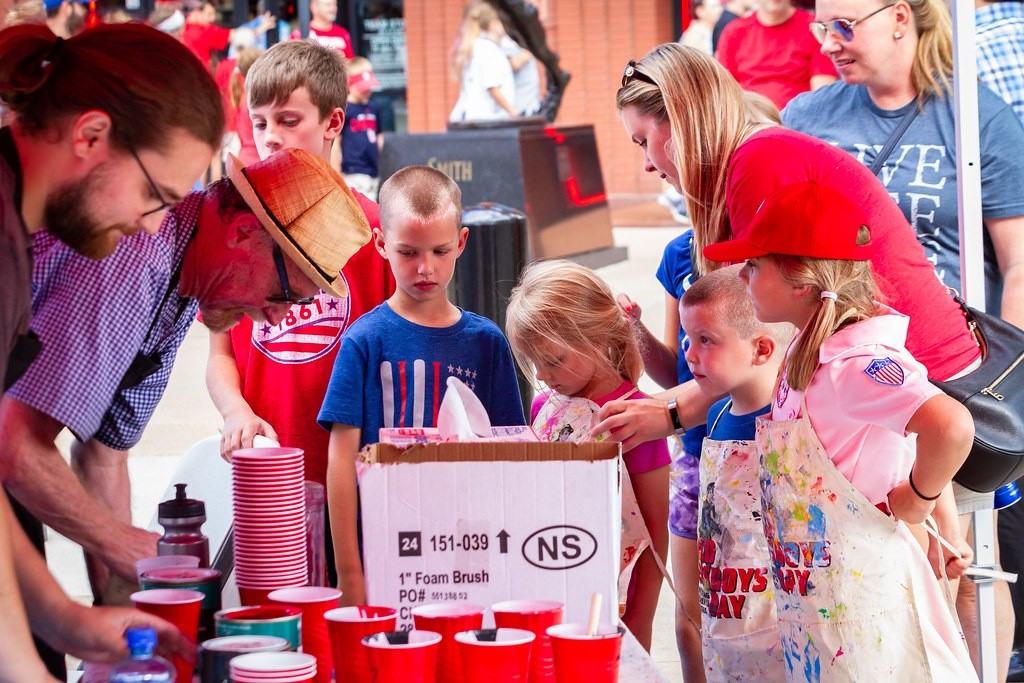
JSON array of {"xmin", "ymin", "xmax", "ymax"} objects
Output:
[
  {"xmin": 666, "ymin": 397, "xmax": 686, "ymax": 437},
  {"xmin": 909, "ymin": 471, "xmax": 941, "ymax": 501}
]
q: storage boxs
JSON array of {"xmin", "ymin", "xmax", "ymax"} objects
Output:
[{"xmin": 354, "ymin": 441, "xmax": 622, "ymax": 627}]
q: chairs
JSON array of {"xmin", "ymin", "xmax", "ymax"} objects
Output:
[{"xmin": 66, "ymin": 434, "xmax": 279, "ymax": 683}]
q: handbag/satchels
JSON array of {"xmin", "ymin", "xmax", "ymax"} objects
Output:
[{"xmin": 928, "ymin": 302, "xmax": 1024, "ymax": 494}]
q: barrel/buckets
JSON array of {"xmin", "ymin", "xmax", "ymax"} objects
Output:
[
  {"xmin": 201, "ymin": 635, "xmax": 290, "ymax": 683},
  {"xmin": 214, "ymin": 606, "xmax": 303, "ymax": 683},
  {"xmin": 139, "ymin": 570, "xmax": 221, "ymax": 683}
]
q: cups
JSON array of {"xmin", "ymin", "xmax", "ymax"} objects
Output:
[
  {"xmin": 232, "ymin": 447, "xmax": 308, "ymax": 607},
  {"xmin": 130, "ymin": 589, "xmax": 205, "ymax": 683},
  {"xmin": 412, "ymin": 603, "xmax": 485, "ymax": 683},
  {"xmin": 361, "ymin": 630, "xmax": 442, "ymax": 683},
  {"xmin": 492, "ymin": 601, "xmax": 565, "ymax": 683},
  {"xmin": 268, "ymin": 587, "xmax": 343, "ymax": 683},
  {"xmin": 546, "ymin": 624, "xmax": 626, "ymax": 683},
  {"xmin": 324, "ymin": 606, "xmax": 399, "ymax": 683},
  {"xmin": 455, "ymin": 628, "xmax": 536, "ymax": 683},
  {"xmin": 135, "ymin": 555, "xmax": 200, "ymax": 591}
]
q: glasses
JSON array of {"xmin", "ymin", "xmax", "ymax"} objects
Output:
[
  {"xmin": 111, "ymin": 126, "xmax": 184, "ymax": 225},
  {"xmin": 269, "ymin": 236, "xmax": 315, "ymax": 308},
  {"xmin": 808, "ymin": 2, "xmax": 896, "ymax": 46},
  {"xmin": 622, "ymin": 61, "xmax": 657, "ymax": 91}
]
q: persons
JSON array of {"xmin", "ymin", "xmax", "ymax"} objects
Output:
[
  {"xmin": 0, "ymin": 0, "xmax": 394, "ymax": 202},
  {"xmin": 316, "ymin": 168, "xmax": 527, "ymax": 612},
  {"xmin": 589, "ymin": 39, "xmax": 995, "ymax": 599},
  {"xmin": 777, "ymin": 0, "xmax": 1024, "ymax": 683},
  {"xmin": 444, "ymin": 0, "xmax": 548, "ymax": 129},
  {"xmin": 674, "ymin": 264, "xmax": 796, "ymax": 683},
  {"xmin": 677, "ymin": 0, "xmax": 841, "ymax": 108},
  {"xmin": 504, "ymin": 256, "xmax": 673, "ymax": 655},
  {"xmin": 0, "ymin": 16, "xmax": 377, "ymax": 683},
  {"xmin": 611, "ymin": 91, "xmax": 784, "ymax": 683},
  {"xmin": 703, "ymin": 179, "xmax": 981, "ymax": 683},
  {"xmin": 196, "ymin": 35, "xmax": 396, "ymax": 589}
]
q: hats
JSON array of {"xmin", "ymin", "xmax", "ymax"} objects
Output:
[
  {"xmin": 224, "ymin": 146, "xmax": 373, "ymax": 299},
  {"xmin": 346, "ymin": 71, "xmax": 380, "ymax": 94},
  {"xmin": 703, "ymin": 174, "xmax": 873, "ymax": 264}
]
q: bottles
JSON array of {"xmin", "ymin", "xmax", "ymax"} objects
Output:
[
  {"xmin": 994, "ymin": 482, "xmax": 1022, "ymax": 510},
  {"xmin": 110, "ymin": 629, "xmax": 175, "ymax": 683},
  {"xmin": 156, "ymin": 484, "xmax": 209, "ymax": 568}
]
